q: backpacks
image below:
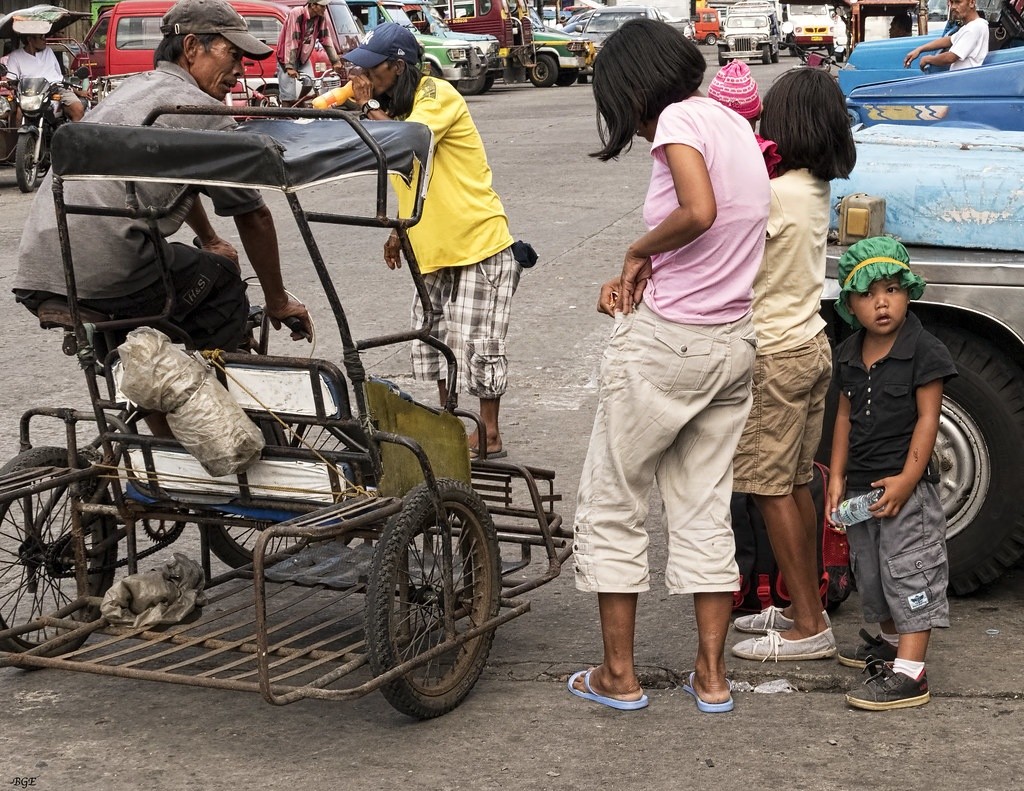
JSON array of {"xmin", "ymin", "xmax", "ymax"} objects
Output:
[{"xmin": 729, "ymin": 462, "xmax": 852, "ymax": 614}]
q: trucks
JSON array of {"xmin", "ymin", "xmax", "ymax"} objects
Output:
[
  {"xmin": 348, "ymin": 0, "xmax": 582, "ymax": 97},
  {"xmin": 665, "ymin": 1, "xmax": 836, "ymax": 66}
]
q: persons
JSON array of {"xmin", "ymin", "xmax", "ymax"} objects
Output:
[
  {"xmin": 340, "ymin": 22, "xmax": 521, "ymax": 460},
  {"xmin": 275, "ymin": 0, "xmax": 343, "ymax": 108},
  {"xmin": 5, "ymin": 34, "xmax": 84, "ymax": 128},
  {"xmin": 903, "ymin": 0, "xmax": 990, "ymax": 74},
  {"xmin": 823, "ymin": 235, "xmax": 959, "ymax": 712},
  {"xmin": 889, "ymin": 14, "xmax": 912, "ymax": 38},
  {"xmin": 567, "ymin": 15, "xmax": 858, "ymax": 715},
  {"xmin": 12, "ymin": 0, "xmax": 315, "ymax": 438}
]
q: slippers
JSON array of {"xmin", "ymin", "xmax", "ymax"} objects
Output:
[
  {"xmin": 469, "ymin": 447, "xmax": 508, "ymax": 461},
  {"xmin": 682, "ymin": 673, "xmax": 734, "ymax": 714},
  {"xmin": 566, "ymin": 666, "xmax": 648, "ymax": 710}
]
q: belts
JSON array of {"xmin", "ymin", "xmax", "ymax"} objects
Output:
[{"xmin": 451, "ymin": 266, "xmax": 462, "ymax": 303}]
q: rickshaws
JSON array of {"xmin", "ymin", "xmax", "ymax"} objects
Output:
[{"xmin": 0, "ymin": 103, "xmax": 574, "ymax": 720}]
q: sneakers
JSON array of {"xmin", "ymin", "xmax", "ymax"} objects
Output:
[
  {"xmin": 731, "ymin": 627, "xmax": 838, "ymax": 660},
  {"xmin": 733, "ymin": 604, "xmax": 831, "ymax": 633},
  {"xmin": 838, "ymin": 628, "xmax": 901, "ymax": 669},
  {"xmin": 846, "ymin": 656, "xmax": 931, "ymax": 710}
]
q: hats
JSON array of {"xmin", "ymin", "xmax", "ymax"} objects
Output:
[
  {"xmin": 340, "ymin": 22, "xmax": 419, "ymax": 69},
  {"xmin": 306, "ymin": 0, "xmax": 333, "ymax": 6},
  {"xmin": 833, "ymin": 234, "xmax": 926, "ymax": 323},
  {"xmin": 160, "ymin": 0, "xmax": 274, "ymax": 62},
  {"xmin": 706, "ymin": 59, "xmax": 761, "ymax": 119}
]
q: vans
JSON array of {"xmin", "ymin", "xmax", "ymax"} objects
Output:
[
  {"xmin": 69, "ymin": 0, "xmax": 366, "ymax": 119},
  {"xmin": 562, "ymin": 6, "xmax": 662, "ymax": 85}
]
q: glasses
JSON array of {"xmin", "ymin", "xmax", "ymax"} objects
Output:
[{"xmin": 30, "ymin": 34, "xmax": 46, "ymax": 40}]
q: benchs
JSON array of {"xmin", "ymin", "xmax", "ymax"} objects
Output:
[{"xmin": 104, "ymin": 352, "xmax": 472, "ymax": 525}]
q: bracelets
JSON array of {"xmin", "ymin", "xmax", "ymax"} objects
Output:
[{"xmin": 389, "ymin": 234, "xmax": 401, "ymax": 239}]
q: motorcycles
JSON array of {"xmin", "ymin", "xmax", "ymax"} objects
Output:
[{"xmin": 0, "ymin": 63, "xmax": 92, "ymax": 191}]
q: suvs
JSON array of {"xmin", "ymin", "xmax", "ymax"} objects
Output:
[{"xmin": 822, "ymin": 30, "xmax": 1024, "ymax": 598}]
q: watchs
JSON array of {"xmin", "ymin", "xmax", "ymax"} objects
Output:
[{"xmin": 364, "ymin": 99, "xmax": 380, "ymax": 115}]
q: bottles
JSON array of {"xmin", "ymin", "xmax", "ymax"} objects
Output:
[
  {"xmin": 831, "ymin": 491, "xmax": 883, "ymax": 525},
  {"xmin": 312, "ymin": 80, "xmax": 355, "ymax": 110}
]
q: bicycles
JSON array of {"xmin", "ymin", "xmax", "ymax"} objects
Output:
[{"xmin": 235, "ymin": 58, "xmax": 333, "ymax": 113}]
